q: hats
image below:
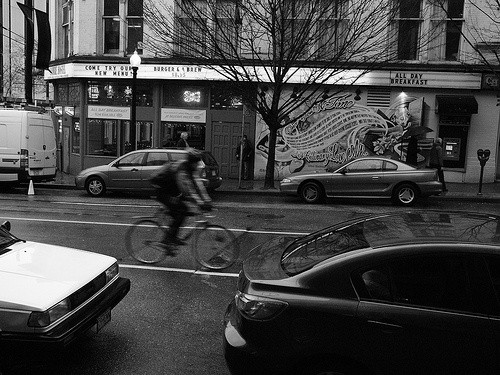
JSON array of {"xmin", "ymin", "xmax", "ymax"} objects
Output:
[{"xmin": 188, "ymin": 152, "xmax": 201, "ymax": 162}]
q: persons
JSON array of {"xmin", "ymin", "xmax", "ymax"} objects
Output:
[
  {"xmin": 235, "ymin": 135, "xmax": 254, "ymax": 180},
  {"xmin": 157, "ymin": 150, "xmax": 214, "ymax": 247},
  {"xmin": 431, "ymin": 138, "xmax": 449, "ymax": 192},
  {"xmin": 175, "ymin": 132, "xmax": 189, "ymax": 147}
]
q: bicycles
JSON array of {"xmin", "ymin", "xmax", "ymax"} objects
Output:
[{"xmin": 124, "ymin": 201, "xmax": 241, "ymax": 271}]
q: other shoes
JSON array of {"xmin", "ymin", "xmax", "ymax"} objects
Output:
[
  {"xmin": 162, "ymin": 241, "xmax": 177, "ymax": 257},
  {"xmin": 174, "ymin": 237, "xmax": 188, "ymax": 246}
]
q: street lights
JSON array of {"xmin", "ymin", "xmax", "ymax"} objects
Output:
[{"xmin": 129, "ymin": 50, "xmax": 142, "ymax": 152}]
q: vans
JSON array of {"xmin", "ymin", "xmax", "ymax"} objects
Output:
[{"xmin": 0, "ymin": 101, "xmax": 57, "ymax": 184}]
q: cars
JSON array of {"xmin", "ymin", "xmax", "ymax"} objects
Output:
[
  {"xmin": 223, "ymin": 207, "xmax": 500, "ymax": 375},
  {"xmin": 279, "ymin": 156, "xmax": 444, "ymax": 208},
  {"xmin": 74, "ymin": 146, "xmax": 223, "ymax": 198},
  {"xmin": 0, "ymin": 219, "xmax": 133, "ymax": 353}
]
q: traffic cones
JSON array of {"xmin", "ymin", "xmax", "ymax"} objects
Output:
[{"xmin": 27, "ymin": 180, "xmax": 35, "ymax": 195}]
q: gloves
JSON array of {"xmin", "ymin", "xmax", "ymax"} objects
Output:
[{"xmin": 200, "ymin": 204, "xmax": 213, "ymax": 212}]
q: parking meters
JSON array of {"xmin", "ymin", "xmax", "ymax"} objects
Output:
[{"xmin": 476, "ymin": 148, "xmax": 491, "ymax": 195}]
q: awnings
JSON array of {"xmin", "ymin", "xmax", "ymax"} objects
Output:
[{"xmin": 435, "ymin": 94, "xmax": 478, "ymax": 115}]
